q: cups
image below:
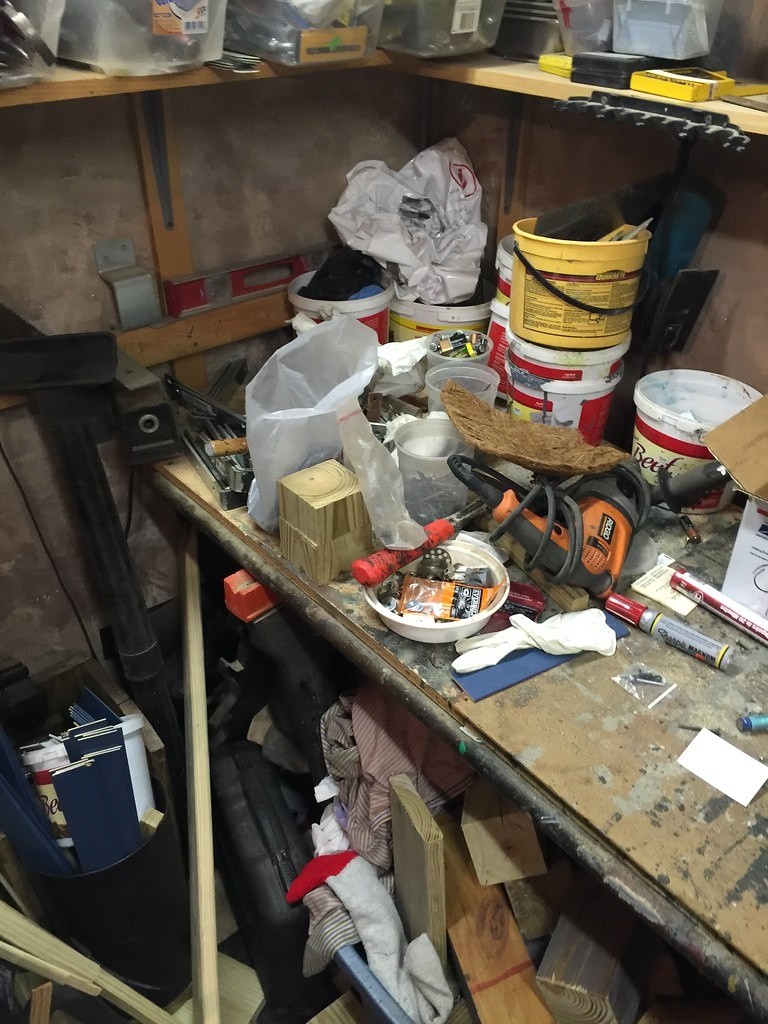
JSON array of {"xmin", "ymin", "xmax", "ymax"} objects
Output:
[
  {"xmin": 424, "ymin": 329, "xmax": 494, "ymax": 370},
  {"xmin": 393, "ymin": 417, "xmax": 475, "ymax": 525},
  {"xmin": 425, "ymin": 360, "xmax": 501, "ymax": 417}
]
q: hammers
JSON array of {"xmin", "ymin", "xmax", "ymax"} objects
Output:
[{"xmin": 351, "ymin": 468, "xmax": 534, "ymax": 589}]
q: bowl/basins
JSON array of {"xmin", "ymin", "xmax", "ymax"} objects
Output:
[{"xmin": 363, "ymin": 540, "xmax": 510, "ymax": 644}]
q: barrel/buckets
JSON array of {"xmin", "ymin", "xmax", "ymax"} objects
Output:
[
  {"xmin": 286, "ymin": 268, "xmax": 395, "ymax": 344},
  {"xmin": 388, "ymin": 277, "xmax": 497, "ymax": 344},
  {"xmin": 486, "ymin": 217, "xmax": 651, "ymax": 443},
  {"xmin": 633, "ymin": 368, "xmax": 766, "ymax": 513},
  {"xmin": 21, "ymin": 712, "xmax": 156, "ymax": 848}
]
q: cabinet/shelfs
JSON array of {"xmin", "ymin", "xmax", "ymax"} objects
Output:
[{"xmin": 0, "ymin": 54, "xmax": 768, "ymax": 1024}]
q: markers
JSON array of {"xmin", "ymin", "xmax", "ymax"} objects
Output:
[
  {"xmin": 669, "ymin": 568, "xmax": 768, "ymax": 645},
  {"xmin": 604, "ymin": 592, "xmax": 735, "ymax": 671},
  {"xmin": 735, "ymin": 715, "xmax": 768, "ymax": 732}
]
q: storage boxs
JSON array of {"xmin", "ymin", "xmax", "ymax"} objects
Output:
[
  {"xmin": 553, "ymin": 0, "xmax": 723, "ymax": 60},
  {"xmin": 376, "ymin": 0, "xmax": 507, "ymax": 58},
  {"xmin": 223, "ymin": 0, "xmax": 384, "ymax": 66},
  {"xmin": 20, "ymin": 0, "xmax": 225, "ymax": 77},
  {"xmin": 702, "ymin": 391, "xmax": 768, "ymax": 620}
]
